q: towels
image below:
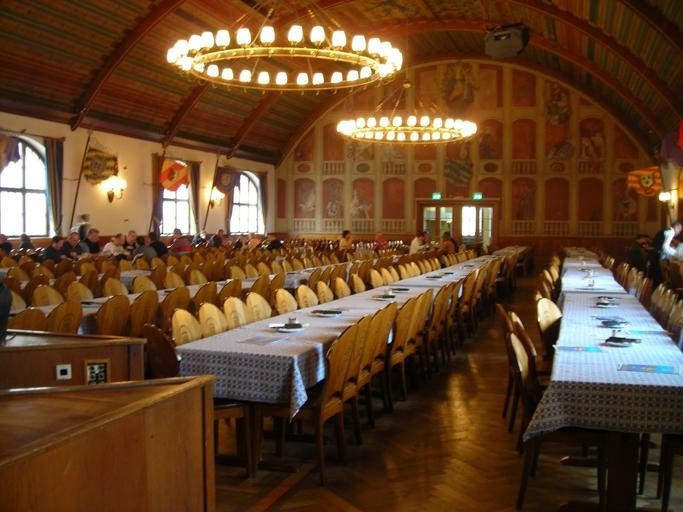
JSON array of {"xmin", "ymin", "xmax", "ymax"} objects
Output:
[
  {"xmin": 268, "ymin": 322, "xmax": 309, "ymax": 328},
  {"xmin": 597, "ymin": 323, "xmax": 628, "ymax": 328},
  {"xmin": 597, "ymin": 304, "xmax": 617, "ymax": 308},
  {"xmin": 392, "ymin": 289, "xmax": 411, "ymax": 293},
  {"xmin": 370, "ymin": 297, "xmax": 393, "ymax": 301},
  {"xmin": 308, "ymin": 311, "xmax": 338, "ymax": 317},
  {"xmin": 80, "ymin": 303, "xmax": 100, "ymax": 308},
  {"xmin": 601, "ymin": 339, "xmax": 632, "ymax": 347}
]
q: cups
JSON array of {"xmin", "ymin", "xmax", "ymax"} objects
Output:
[
  {"xmin": 587, "ymin": 269, "xmax": 594, "ymax": 278},
  {"xmin": 384, "ymin": 288, "xmax": 392, "ymax": 295},
  {"xmin": 288, "ymin": 316, "xmax": 297, "ymax": 326}
]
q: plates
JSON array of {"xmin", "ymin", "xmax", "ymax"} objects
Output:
[
  {"xmin": 310, "ymin": 309, "xmax": 342, "ymax": 318},
  {"xmin": 369, "ymin": 294, "xmax": 395, "ymax": 301},
  {"xmin": 393, "ymin": 287, "xmax": 410, "ymax": 293},
  {"xmin": 598, "ymin": 295, "xmax": 617, "ymax": 301},
  {"xmin": 278, "ymin": 325, "xmax": 303, "ymax": 332},
  {"xmin": 81, "ymin": 300, "xmax": 104, "ymax": 308},
  {"xmin": 426, "ymin": 276, "xmax": 442, "ymax": 280},
  {"xmin": 596, "ymin": 302, "xmax": 618, "ymax": 307},
  {"xmin": 605, "ymin": 337, "xmax": 632, "ymax": 347},
  {"xmin": 603, "ymin": 318, "xmax": 629, "ymax": 336}
]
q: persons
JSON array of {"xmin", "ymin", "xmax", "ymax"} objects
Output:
[
  {"xmin": 267, "ymin": 233, "xmax": 284, "ymax": 250},
  {"xmin": 0, "ymin": 242, "xmax": 12, "ymax": 257},
  {"xmin": 133, "ymin": 236, "xmax": 157, "ymax": 260},
  {"xmin": 148, "ymin": 232, "xmax": 169, "ymax": 261},
  {"xmin": 43, "ymin": 236, "xmax": 63, "ymax": 262},
  {"xmin": 0, "ymin": 234, "xmax": 8, "ymax": 243},
  {"xmin": 207, "ymin": 230, "xmax": 224, "ymax": 247},
  {"xmin": 125, "ymin": 230, "xmax": 136, "ymax": 254},
  {"xmin": 192, "ymin": 231, "xmax": 210, "ymax": 249},
  {"xmin": 339, "ymin": 230, "xmax": 353, "ymax": 252},
  {"xmin": 233, "ymin": 234, "xmax": 245, "ymax": 250},
  {"xmin": 103, "ymin": 234, "xmax": 129, "ymax": 261},
  {"xmin": 409, "ymin": 231, "xmax": 431, "ymax": 254},
  {"xmin": 439, "ymin": 232, "xmax": 458, "ymax": 254},
  {"xmin": 71, "ymin": 215, "xmax": 91, "ymax": 239},
  {"xmin": 171, "ymin": 229, "xmax": 193, "ymax": 254},
  {"xmin": 64, "ymin": 232, "xmax": 84, "ymax": 259},
  {"xmin": 80, "ymin": 229, "xmax": 100, "ymax": 254},
  {"xmin": 648, "ymin": 222, "xmax": 683, "ymax": 271},
  {"xmin": 373, "ymin": 233, "xmax": 389, "ymax": 251},
  {"xmin": 633, "ymin": 233, "xmax": 647, "ymax": 262},
  {"xmin": 17, "ymin": 234, "xmax": 34, "ymax": 249}
]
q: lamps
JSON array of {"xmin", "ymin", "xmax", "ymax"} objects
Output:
[
  {"xmin": 102, "ymin": 175, "xmax": 126, "ymax": 203},
  {"xmin": 211, "ymin": 185, "xmax": 225, "ymax": 208},
  {"xmin": 336, "ymin": 25, "xmax": 479, "ymax": 145},
  {"xmin": 658, "ymin": 189, "xmax": 676, "ymax": 203},
  {"xmin": 166, "ymin": 2, "xmax": 402, "ymax": 95}
]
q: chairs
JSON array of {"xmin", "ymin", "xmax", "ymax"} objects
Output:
[
  {"xmin": 329, "ymin": 255, "xmax": 339, "ymax": 264},
  {"xmin": 34, "ymin": 287, "xmax": 64, "ymax": 307},
  {"xmin": 129, "ymin": 290, "xmax": 160, "ymax": 337},
  {"xmin": 534, "ymin": 291, "xmax": 543, "ymax": 304},
  {"xmin": 2, "ymin": 256, "xmax": 17, "ymax": 268},
  {"xmin": 180, "ymin": 255, "xmax": 193, "ymax": 265},
  {"xmin": 133, "ymin": 276, "xmax": 157, "ymax": 294},
  {"xmin": 80, "ymin": 262, "xmax": 98, "ymax": 277},
  {"xmin": 456, "ymin": 271, "xmax": 476, "ymax": 356},
  {"xmin": 271, "ymin": 272, "xmax": 286, "ymax": 308},
  {"xmin": 352, "ymin": 274, "xmax": 366, "ymax": 294},
  {"xmin": 79, "ymin": 271, "xmax": 98, "ymax": 298},
  {"xmin": 160, "ymin": 287, "xmax": 190, "ymax": 332},
  {"xmin": 588, "ymin": 246, "xmax": 638, "ymax": 291},
  {"xmin": 317, "ymin": 281, "xmax": 334, "ymax": 304},
  {"xmin": 442, "ymin": 249, "xmax": 477, "ymax": 267},
  {"xmin": 341, "ymin": 264, "xmax": 347, "ymax": 282},
  {"xmin": 398, "ymin": 264, "xmax": 408, "ymax": 280},
  {"xmin": 7, "ymin": 309, "xmax": 47, "ymax": 331},
  {"xmin": 536, "ymin": 298, "xmax": 562, "ymax": 362},
  {"xmin": 331, "ymin": 265, "xmax": 341, "ymax": 290},
  {"xmin": 658, "ymin": 294, "xmax": 678, "ymax": 330},
  {"xmin": 170, "ymin": 264, "xmax": 185, "ymax": 284},
  {"xmin": 41, "ymin": 259, "xmax": 56, "ymax": 272},
  {"xmin": 99, "ymin": 269, "xmax": 120, "ymax": 297},
  {"xmin": 421, "ymin": 285, "xmax": 448, "ymax": 377},
  {"xmin": 502, "ymin": 310, "xmax": 553, "ymax": 435},
  {"xmin": 507, "ymin": 332, "xmax": 615, "ymax": 512},
  {"xmin": 312, "ymin": 256, "xmax": 323, "ymax": 267},
  {"xmin": 296, "ymin": 285, "xmax": 319, "ymax": 309},
  {"xmin": 425, "ymin": 288, "xmax": 434, "ymax": 396},
  {"xmin": 218, "ymin": 279, "xmax": 242, "ymax": 309},
  {"xmin": 225, "ymin": 248, "xmax": 316, "ymax": 280},
  {"xmin": 105, "ymin": 277, "xmax": 128, "ymax": 296},
  {"xmin": 271, "ymin": 260, "xmax": 283, "ymax": 275},
  {"xmin": 192, "ymin": 281, "xmax": 218, "ymax": 317},
  {"xmin": 321, "ymin": 266, "xmax": 331, "ymax": 286},
  {"xmin": 170, "ymin": 309, "xmax": 202, "ymax": 346},
  {"xmin": 303, "ymin": 258, "xmax": 312, "ymax": 269},
  {"xmin": 429, "ymin": 258, "xmax": 436, "ymax": 271},
  {"xmin": 495, "ymin": 304, "xmax": 551, "ymax": 458},
  {"xmin": 292, "ymin": 258, "xmax": 304, "ymax": 271},
  {"xmin": 67, "ymin": 282, "xmax": 93, "ymax": 301},
  {"xmin": 193, "ymin": 252, "xmax": 205, "ymax": 266},
  {"xmin": 549, "ymin": 255, "xmax": 561, "ymax": 287},
  {"xmin": 256, "ymin": 262, "xmax": 271, "ymax": 275},
  {"xmin": 359, "ymin": 262, "xmax": 368, "ymax": 284},
  {"xmin": 229, "ymin": 266, "xmax": 246, "ymax": 280},
  {"xmin": 149, "ymin": 264, "xmax": 167, "ymax": 290},
  {"xmin": 309, "ymin": 268, "xmax": 322, "ymax": 292},
  {"xmin": 119, "ymin": 259, "xmax": 133, "ymax": 272},
  {"xmin": 434, "ymin": 257, "xmax": 441, "ymax": 269},
  {"xmin": 53, "ymin": 272, "xmax": 76, "ymax": 300},
  {"xmin": 101, "ymin": 261, "xmax": 118, "ymax": 273},
  {"xmin": 423, "ymin": 259, "xmax": 432, "ymax": 273},
  {"xmin": 188, "ymin": 270, "xmax": 208, "ymax": 285},
  {"xmin": 346, "ymin": 248, "xmax": 400, "ymax": 262},
  {"xmin": 166, "ymin": 255, "xmax": 180, "ymax": 266},
  {"xmin": 56, "ymin": 256, "xmax": 110, "ymax": 279},
  {"xmin": 335, "ymin": 277, "xmax": 351, "ymax": 299},
  {"xmin": 540, "ymin": 280, "xmax": 553, "ymax": 302},
  {"xmin": 471, "ymin": 269, "xmax": 486, "ymax": 338},
  {"xmin": 249, "ymin": 274, "xmax": 271, "ymax": 303},
  {"xmin": 663, "ymin": 300, "xmax": 683, "ymax": 343},
  {"xmin": 417, "ymin": 260, "xmax": 426, "ymax": 275},
  {"xmin": 135, "ymin": 259, "xmax": 149, "ymax": 270},
  {"xmin": 22, "ymin": 274, "xmax": 49, "ymax": 307},
  {"xmin": 349, "ymin": 263, "xmax": 359, "ymax": 287},
  {"xmin": 47, "ymin": 301, "xmax": 83, "ymax": 334},
  {"xmin": 199, "ymin": 303, "xmax": 228, "ymax": 338},
  {"xmin": 18, "ymin": 262, "xmax": 36, "ymax": 276},
  {"xmin": 484, "ymin": 260, "xmax": 500, "ymax": 319},
  {"xmin": 439, "ymin": 282, "xmax": 455, "ymax": 373},
  {"xmin": 389, "ymin": 266, "xmax": 399, "ymax": 283},
  {"xmin": 277, "ymin": 230, "xmax": 403, "ymax": 256},
  {"xmin": 282, "ymin": 259, "xmax": 293, "ymax": 272},
  {"xmin": 274, "ymin": 288, "xmax": 298, "ymax": 315},
  {"xmin": 671, "ymin": 317, "xmax": 682, "ymax": 349},
  {"xmin": 645, "ymin": 283, "xmax": 663, "ymax": 313},
  {"xmin": 383, "ymin": 302, "xmax": 399, "ymax": 413},
  {"xmin": 381, "ymin": 267, "xmax": 393, "ymax": 286},
  {"xmin": 200, "ymin": 259, "xmax": 215, "ymax": 282},
  {"xmin": 368, "ymin": 309, "xmax": 391, "ymax": 429},
  {"xmin": 185, "ymin": 262, "xmax": 202, "ymax": 286},
  {"xmin": 446, "ymin": 279, "xmax": 464, "ymax": 367},
  {"xmin": 404, "ymin": 262, "xmax": 414, "ymax": 278},
  {"xmin": 634, "ymin": 278, "xmax": 649, "ymax": 303},
  {"xmin": 139, "ymin": 323, "xmax": 253, "ymax": 478},
  {"xmin": 496, "ymin": 250, "xmax": 532, "ymax": 303},
  {"xmin": 245, "ymin": 264, "xmax": 258, "ymax": 278},
  {"xmin": 10, "ymin": 288, "xmax": 26, "ymax": 309},
  {"xmin": 31, "ymin": 266, "xmax": 55, "ymax": 281},
  {"xmin": 390, "ymin": 298, "xmax": 418, "ymax": 412},
  {"xmin": 151, "ymin": 257, "xmax": 166, "ymax": 270},
  {"xmin": 629, "ymin": 271, "xmax": 643, "ymax": 295},
  {"xmin": 7, "ymin": 268, "xmax": 30, "ymax": 290},
  {"xmin": 543, "ymin": 269, "xmax": 558, "ymax": 291},
  {"xmin": 652, "ymin": 289, "xmax": 673, "ymax": 323},
  {"xmin": 374, "ymin": 253, "xmax": 439, "ymax": 269},
  {"xmin": 223, "ymin": 297, "xmax": 253, "ymax": 331},
  {"xmin": 289, "ymin": 314, "xmax": 373, "ymax": 463},
  {"xmin": 163, "ymin": 272, "xmax": 185, "ymax": 289},
  {"xmin": 322, "ymin": 255, "xmax": 331, "ymax": 265},
  {"xmin": 648, "ymin": 287, "xmax": 665, "ymax": 318},
  {"xmin": 253, "ymin": 325, "xmax": 359, "ymax": 485},
  {"xmin": 369, "ymin": 269, "xmax": 383, "ymax": 289},
  {"xmin": 96, "ymin": 295, "xmax": 130, "ymax": 335},
  {"xmin": 367, "ymin": 260, "xmax": 373, "ymax": 284},
  {"xmin": 410, "ymin": 262, "xmax": 421, "ymax": 276},
  {"xmin": 404, "ymin": 294, "xmax": 428, "ymax": 401},
  {"xmin": 214, "ymin": 254, "xmax": 225, "ymax": 282},
  {"xmin": 247, "ymin": 292, "xmax": 272, "ymax": 323}
]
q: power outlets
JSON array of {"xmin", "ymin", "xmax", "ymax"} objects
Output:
[{"xmin": 55, "ymin": 364, "xmax": 72, "ymax": 381}]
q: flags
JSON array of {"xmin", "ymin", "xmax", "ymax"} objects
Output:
[
  {"xmin": 70, "ymin": 129, "xmax": 118, "ymax": 229},
  {"xmin": 146, "ymin": 148, "xmax": 192, "ymax": 234},
  {"xmin": 623, "ymin": 164, "xmax": 673, "ymax": 229},
  {"xmin": 204, "ymin": 154, "xmax": 241, "ymax": 233}
]
